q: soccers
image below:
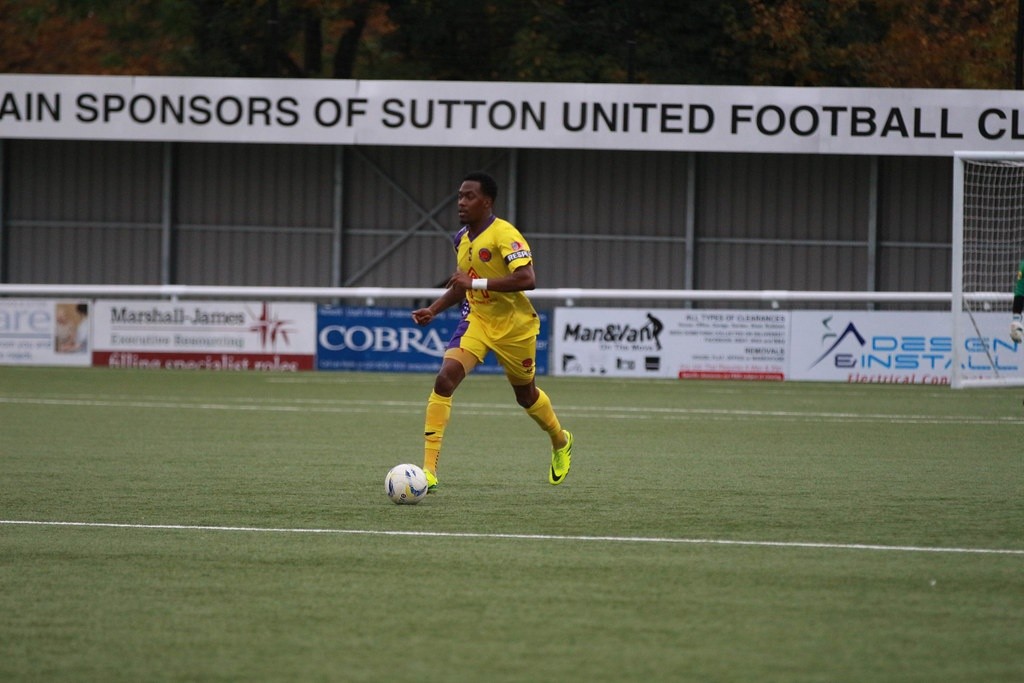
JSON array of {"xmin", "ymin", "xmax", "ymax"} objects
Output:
[{"xmin": 385, "ymin": 463, "xmax": 428, "ymax": 505}]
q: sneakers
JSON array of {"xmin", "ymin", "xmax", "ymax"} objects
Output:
[
  {"xmin": 549, "ymin": 430, "xmax": 574, "ymax": 484},
  {"xmin": 422, "ymin": 468, "xmax": 438, "ymax": 492}
]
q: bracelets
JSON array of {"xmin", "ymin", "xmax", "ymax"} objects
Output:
[{"xmin": 472, "ymin": 278, "xmax": 488, "ymax": 290}]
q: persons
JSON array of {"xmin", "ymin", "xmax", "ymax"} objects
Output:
[
  {"xmin": 56, "ymin": 303, "xmax": 88, "ymax": 353},
  {"xmin": 1010, "ymin": 259, "xmax": 1024, "ymax": 344},
  {"xmin": 411, "ymin": 174, "xmax": 573, "ymax": 493}
]
